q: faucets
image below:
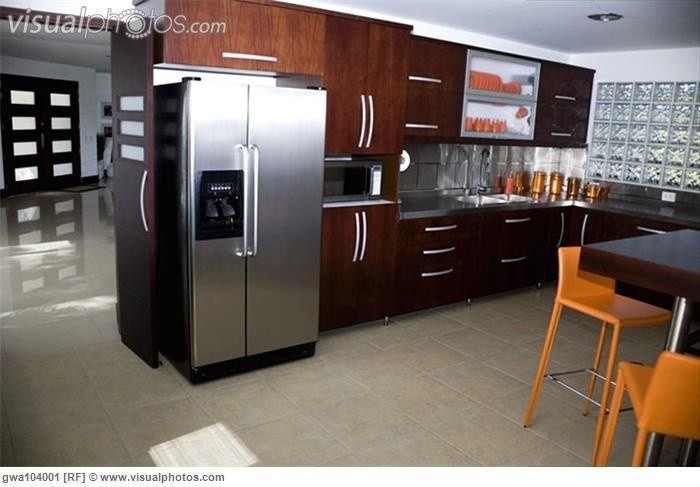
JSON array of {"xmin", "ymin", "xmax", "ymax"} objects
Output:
[
  {"xmin": 461, "ymin": 159, "xmax": 470, "ymax": 195},
  {"xmin": 477, "ymin": 147, "xmax": 492, "ymax": 193}
]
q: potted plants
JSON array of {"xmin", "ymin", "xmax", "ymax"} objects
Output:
[{"xmin": 96, "ymin": 131, "xmax": 106, "ymax": 180}]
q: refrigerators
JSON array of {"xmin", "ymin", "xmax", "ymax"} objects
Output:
[{"xmin": 151, "ymin": 76, "xmax": 327, "ymax": 387}]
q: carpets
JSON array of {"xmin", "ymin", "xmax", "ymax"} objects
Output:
[{"xmin": 54, "ymin": 183, "xmax": 106, "ymax": 193}]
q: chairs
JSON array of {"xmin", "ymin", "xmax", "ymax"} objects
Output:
[
  {"xmin": 523, "ymin": 247, "xmax": 671, "ymax": 466},
  {"xmin": 595, "ymin": 351, "xmax": 700, "ymax": 466}
]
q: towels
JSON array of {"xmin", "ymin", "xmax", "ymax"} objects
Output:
[{"xmin": 147, "ymin": 420, "xmax": 261, "ymax": 467}]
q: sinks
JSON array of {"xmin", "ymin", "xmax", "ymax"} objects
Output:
[
  {"xmin": 484, "ymin": 192, "xmax": 533, "ymax": 203},
  {"xmin": 454, "ymin": 195, "xmax": 505, "ymax": 205}
]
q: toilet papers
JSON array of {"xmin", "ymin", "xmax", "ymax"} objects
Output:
[{"xmin": 400, "ymin": 150, "xmax": 412, "ymax": 173}]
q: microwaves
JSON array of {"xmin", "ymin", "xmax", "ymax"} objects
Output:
[{"xmin": 323, "ymin": 161, "xmax": 383, "ymax": 205}]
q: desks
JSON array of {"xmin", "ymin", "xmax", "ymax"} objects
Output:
[{"xmin": 584, "ymin": 228, "xmax": 699, "ymax": 466}]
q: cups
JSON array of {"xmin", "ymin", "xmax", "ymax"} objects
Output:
[{"xmin": 516, "ymin": 105, "xmax": 528, "ymax": 119}]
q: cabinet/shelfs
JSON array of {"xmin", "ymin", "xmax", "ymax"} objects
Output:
[{"xmin": 133, "ymin": 0, "xmax": 700, "ymax": 334}]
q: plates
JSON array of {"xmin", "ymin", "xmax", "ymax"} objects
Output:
[{"xmin": 469, "ymin": 70, "xmax": 521, "ymax": 96}]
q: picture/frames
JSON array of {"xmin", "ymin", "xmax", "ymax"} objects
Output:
[
  {"xmin": 102, "ymin": 102, "xmax": 114, "ymax": 119},
  {"xmin": 100, "ymin": 123, "xmax": 114, "ymax": 139}
]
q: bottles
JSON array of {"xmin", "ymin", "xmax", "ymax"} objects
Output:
[
  {"xmin": 494, "ymin": 171, "xmax": 601, "ymax": 199},
  {"xmin": 464, "ymin": 117, "xmax": 507, "ymax": 134}
]
